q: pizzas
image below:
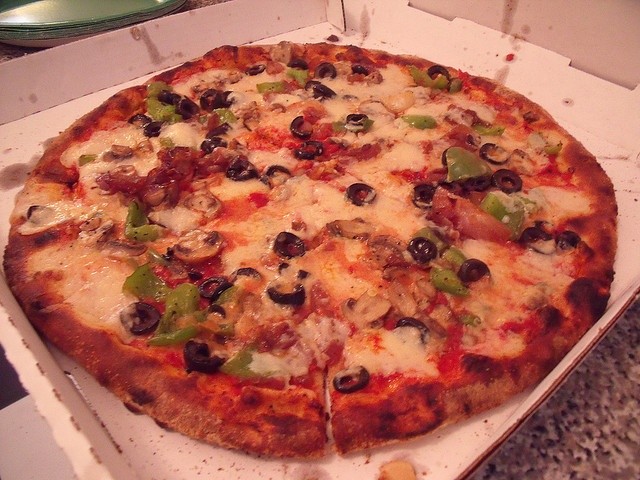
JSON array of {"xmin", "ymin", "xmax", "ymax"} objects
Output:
[{"xmin": 2, "ymin": 40, "xmax": 618, "ymax": 458}]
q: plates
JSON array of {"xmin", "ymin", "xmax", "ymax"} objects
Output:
[{"xmin": 0, "ymin": 1, "xmax": 188, "ymax": 48}]
